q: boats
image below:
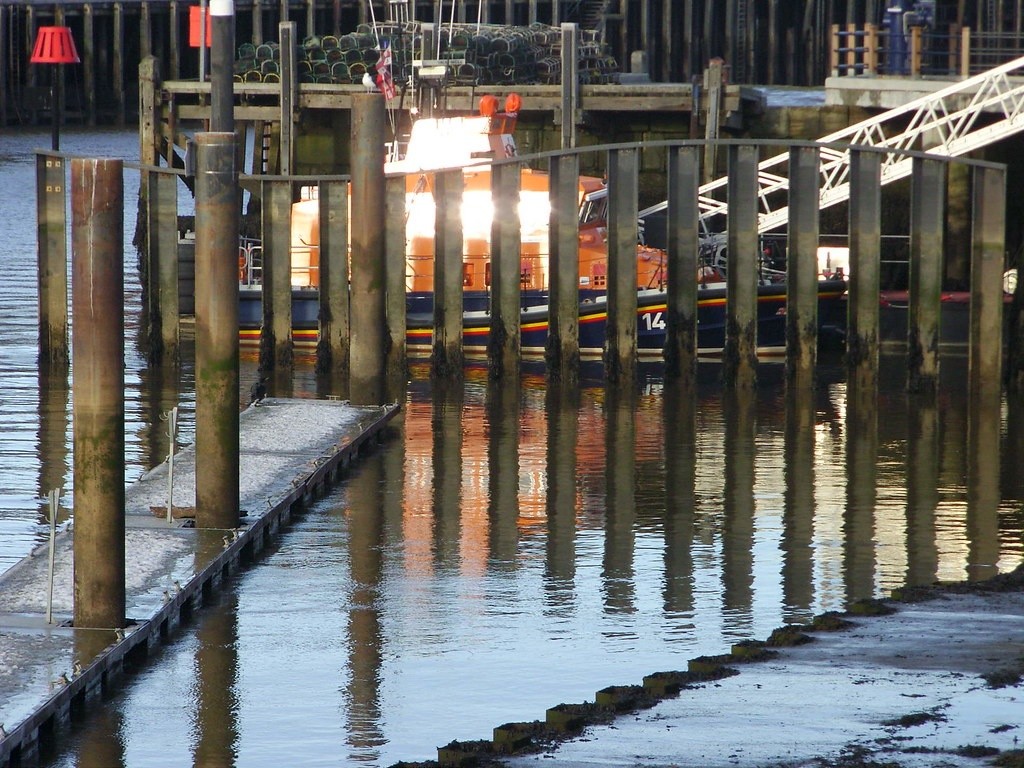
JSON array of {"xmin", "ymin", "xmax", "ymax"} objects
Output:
[{"xmin": 222, "ymin": 94, "xmax": 1024, "ymax": 371}]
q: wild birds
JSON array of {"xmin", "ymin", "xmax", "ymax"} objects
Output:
[{"xmin": 362, "ymin": 72, "xmax": 376, "ymax": 95}]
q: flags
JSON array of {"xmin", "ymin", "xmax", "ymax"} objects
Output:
[{"xmin": 375, "ymin": 43, "xmax": 395, "ymax": 97}]
